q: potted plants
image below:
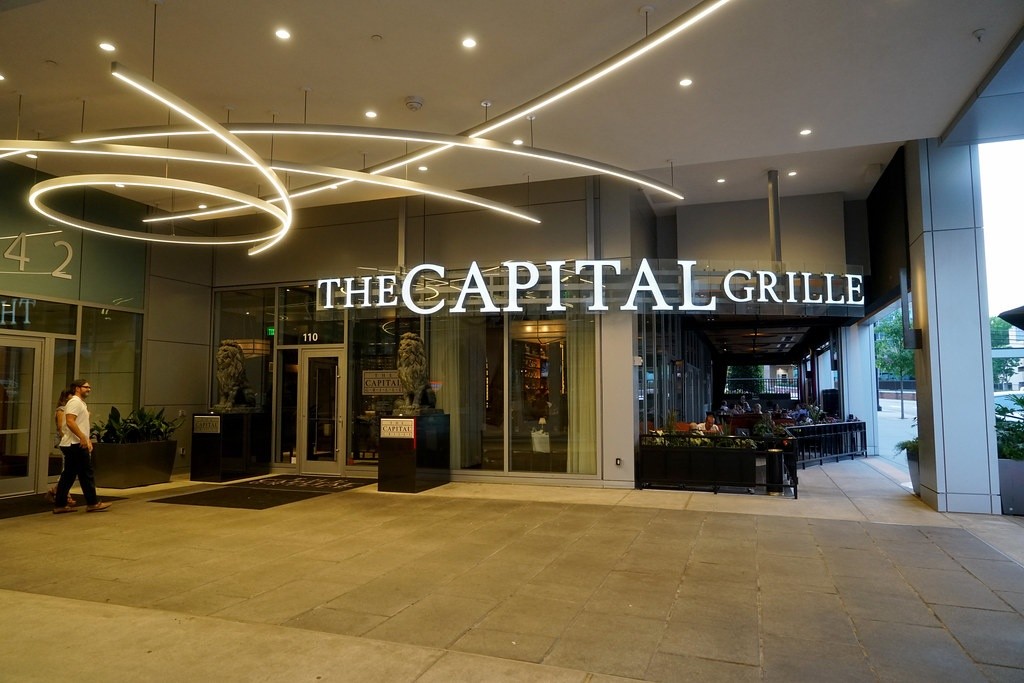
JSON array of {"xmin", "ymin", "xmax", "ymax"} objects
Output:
[
  {"xmin": 90, "ymin": 407, "xmax": 185, "ymax": 488},
  {"xmin": 893, "ymin": 391, "xmax": 1024, "ymax": 516}
]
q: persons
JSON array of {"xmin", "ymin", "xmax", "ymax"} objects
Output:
[
  {"xmin": 531, "ymin": 393, "xmax": 548, "ymax": 419},
  {"xmin": 774, "ymin": 403, "xmax": 791, "ymax": 418},
  {"xmin": 52, "ymin": 378, "xmax": 112, "ymax": 514},
  {"xmin": 697, "ymin": 415, "xmax": 719, "ymax": 431},
  {"xmin": 720, "ymin": 395, "xmax": 762, "ymax": 415},
  {"xmin": 686, "ymin": 422, "xmax": 704, "ymax": 446},
  {"xmin": 44, "ymin": 391, "xmax": 76, "ymax": 505},
  {"xmin": 762, "ymin": 411, "xmax": 775, "ymax": 426},
  {"xmin": 795, "ymin": 404, "xmax": 813, "ymax": 426}
]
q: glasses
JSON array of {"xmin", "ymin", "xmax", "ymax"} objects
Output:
[{"xmin": 81, "ymin": 386, "xmax": 90, "ymax": 389}]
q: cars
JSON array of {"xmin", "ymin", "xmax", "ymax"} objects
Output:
[{"xmin": 994, "ymin": 391, "xmax": 1024, "ymax": 427}]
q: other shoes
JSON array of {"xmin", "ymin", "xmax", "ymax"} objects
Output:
[
  {"xmin": 66, "ymin": 498, "xmax": 76, "ymax": 505},
  {"xmin": 44, "ymin": 490, "xmax": 58, "ymax": 502},
  {"xmin": 87, "ymin": 502, "xmax": 112, "ymax": 512},
  {"xmin": 54, "ymin": 507, "xmax": 78, "ymax": 514}
]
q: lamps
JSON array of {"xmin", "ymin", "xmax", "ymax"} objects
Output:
[
  {"xmin": 0, "ymin": 0, "xmax": 730, "ymax": 256},
  {"xmin": 538, "ymin": 417, "xmax": 547, "ymax": 429}
]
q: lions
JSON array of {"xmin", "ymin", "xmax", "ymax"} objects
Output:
[
  {"xmin": 216, "ymin": 340, "xmax": 256, "ymax": 410},
  {"xmin": 394, "ymin": 332, "xmax": 437, "ymax": 411}
]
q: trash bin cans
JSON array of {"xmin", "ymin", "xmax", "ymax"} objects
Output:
[{"xmin": 766, "ymin": 448, "xmax": 783, "ymax": 495}]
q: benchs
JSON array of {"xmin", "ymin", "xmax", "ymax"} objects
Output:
[{"xmin": 730, "ymin": 413, "xmax": 796, "ymax": 436}]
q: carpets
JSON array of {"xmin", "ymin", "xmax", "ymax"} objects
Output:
[
  {"xmin": 145, "ymin": 474, "xmax": 378, "ymax": 510},
  {"xmin": 0, "ymin": 490, "xmax": 129, "ymax": 520}
]
